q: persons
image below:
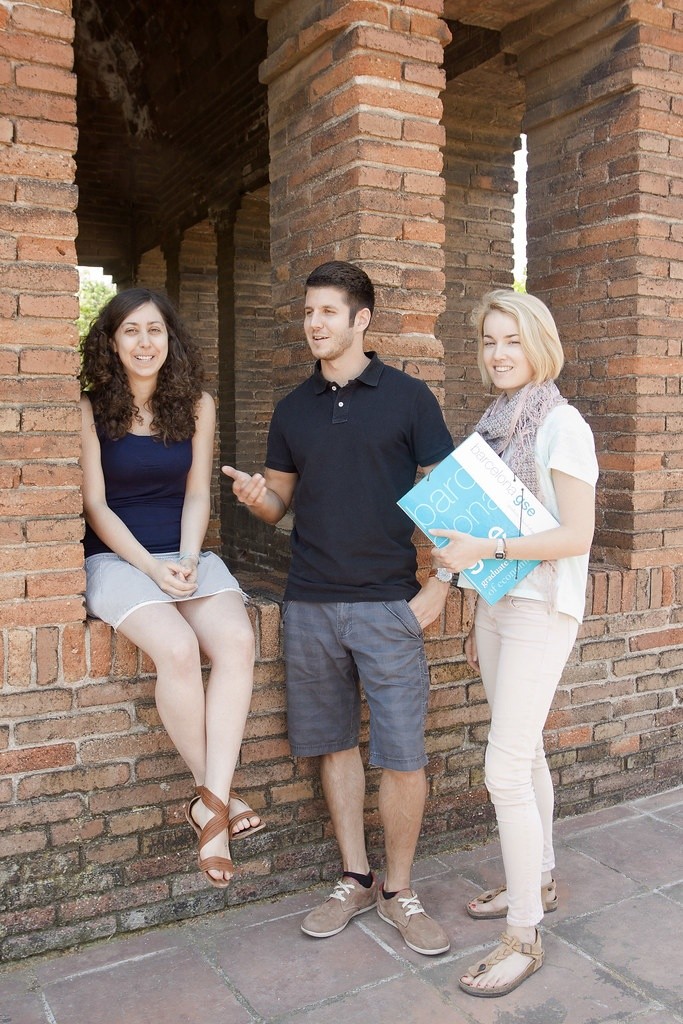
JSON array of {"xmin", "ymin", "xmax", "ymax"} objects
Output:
[
  {"xmin": 78, "ymin": 290, "xmax": 266, "ymax": 887},
  {"xmin": 428, "ymin": 290, "xmax": 599, "ymax": 996},
  {"xmin": 221, "ymin": 260, "xmax": 452, "ymax": 954}
]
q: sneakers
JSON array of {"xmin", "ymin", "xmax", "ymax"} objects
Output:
[
  {"xmin": 376, "ymin": 883, "xmax": 451, "ymax": 955},
  {"xmin": 300, "ymin": 873, "xmax": 380, "ymax": 938}
]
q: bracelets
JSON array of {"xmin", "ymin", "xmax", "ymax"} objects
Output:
[
  {"xmin": 502, "ymin": 538, "xmax": 507, "ymax": 560},
  {"xmin": 180, "ymin": 552, "xmax": 201, "ymax": 564}
]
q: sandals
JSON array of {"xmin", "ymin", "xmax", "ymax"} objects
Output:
[
  {"xmin": 468, "ymin": 874, "xmax": 559, "ymax": 919},
  {"xmin": 184, "ymin": 787, "xmax": 231, "ymax": 888},
  {"xmin": 194, "ymin": 783, "xmax": 266, "ymax": 841},
  {"xmin": 459, "ymin": 932, "xmax": 545, "ymax": 998}
]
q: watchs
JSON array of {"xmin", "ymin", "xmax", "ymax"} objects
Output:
[
  {"xmin": 428, "ymin": 567, "xmax": 454, "ymax": 584},
  {"xmin": 494, "ymin": 539, "xmax": 505, "ymax": 560}
]
